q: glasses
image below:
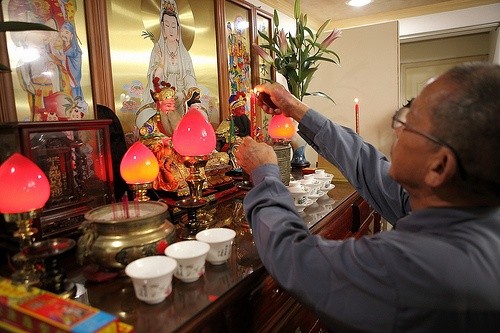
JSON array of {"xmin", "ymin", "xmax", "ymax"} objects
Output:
[{"xmin": 391, "ymin": 98, "xmax": 460, "ymax": 168}]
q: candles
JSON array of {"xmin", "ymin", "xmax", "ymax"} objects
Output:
[
  {"xmin": 355, "ymin": 99, "xmax": 360, "ymax": 135},
  {"xmin": 251, "ymin": 91, "xmax": 255, "ymax": 141}
]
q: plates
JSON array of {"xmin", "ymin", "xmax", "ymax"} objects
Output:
[
  {"xmin": 294, "ymin": 199, "xmax": 313, "ymax": 212},
  {"xmin": 320, "ymin": 184, "xmax": 335, "ymax": 194},
  {"xmin": 309, "ymin": 192, "xmax": 324, "ymax": 202}
]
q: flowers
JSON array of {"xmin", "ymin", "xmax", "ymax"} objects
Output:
[{"xmin": 251, "ymin": 1, "xmax": 343, "ymax": 102}]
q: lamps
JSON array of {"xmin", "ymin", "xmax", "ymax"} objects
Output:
[
  {"xmin": 120, "ymin": 141, "xmax": 159, "ymax": 202},
  {"xmin": 268, "ymin": 113, "xmax": 295, "ymax": 146},
  {"xmin": 0, "ymin": 153, "xmax": 50, "ymax": 285},
  {"xmin": 172, "ymin": 106, "xmax": 216, "ymax": 231}
]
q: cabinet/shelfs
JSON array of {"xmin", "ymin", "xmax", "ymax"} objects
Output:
[{"xmin": 71, "ymin": 181, "xmax": 382, "ymax": 332}]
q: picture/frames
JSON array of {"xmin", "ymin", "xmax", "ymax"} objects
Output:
[{"xmin": 0, "ymin": 0, "xmax": 276, "ymax": 142}]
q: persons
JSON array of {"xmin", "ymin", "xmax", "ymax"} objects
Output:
[
  {"xmin": 138, "ymin": 77, "xmax": 206, "ymax": 192},
  {"xmin": 216, "ymin": 90, "xmax": 252, "ymax": 170},
  {"xmin": 233, "ymin": 62, "xmax": 499, "ymax": 330},
  {"xmin": 181, "ymin": 85, "xmax": 230, "ymax": 167}
]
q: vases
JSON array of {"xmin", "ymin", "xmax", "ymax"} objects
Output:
[{"xmin": 289, "ymin": 118, "xmax": 311, "ymax": 169}]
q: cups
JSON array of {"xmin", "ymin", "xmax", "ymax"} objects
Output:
[
  {"xmin": 164, "ymin": 240, "xmax": 211, "ymax": 282},
  {"xmin": 196, "ymin": 228, "xmax": 236, "ymax": 264},
  {"xmin": 297, "ymin": 175, "xmax": 324, "ymax": 195},
  {"xmin": 125, "ymin": 255, "xmax": 177, "ymax": 304},
  {"xmin": 310, "ymin": 169, "xmax": 334, "ymax": 188},
  {"xmin": 285, "ymin": 181, "xmax": 310, "ymax": 204}
]
